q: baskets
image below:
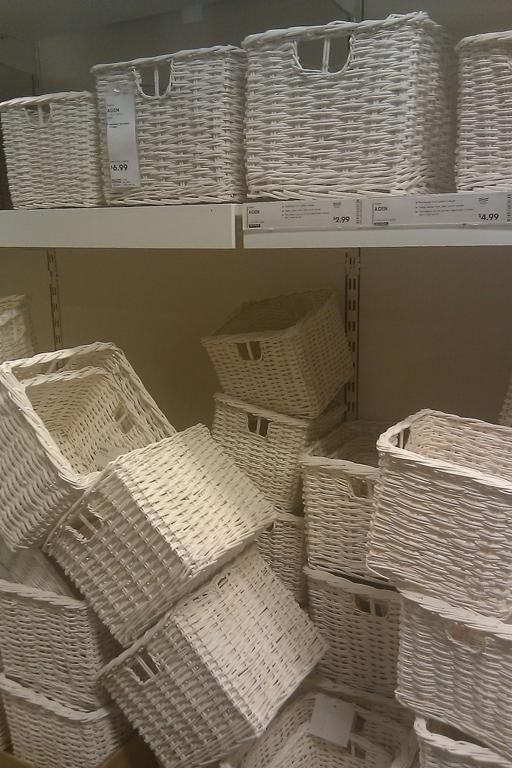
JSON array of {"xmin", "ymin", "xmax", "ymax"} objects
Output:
[
  {"xmin": 0, "ymin": 282, "xmax": 512, "ymax": 767},
  {"xmin": 0, "ymin": 16, "xmax": 512, "ymax": 210}
]
